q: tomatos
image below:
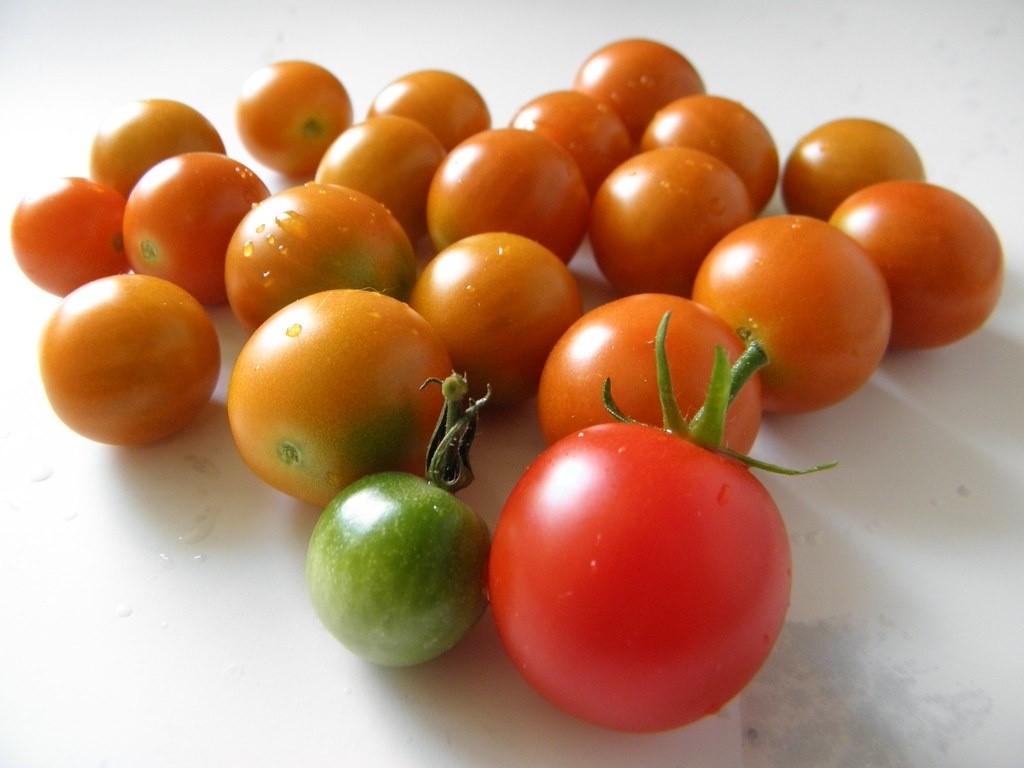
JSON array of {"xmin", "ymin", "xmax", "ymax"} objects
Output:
[{"xmin": 10, "ymin": 37, "xmax": 1002, "ymax": 733}]
